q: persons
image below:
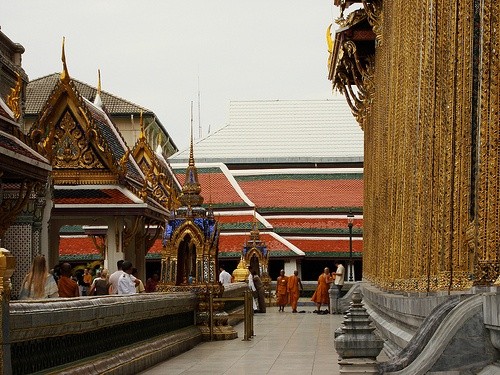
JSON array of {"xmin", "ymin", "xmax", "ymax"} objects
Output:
[
  {"xmin": 18, "ymin": 254, "xmax": 59, "ymax": 300},
  {"xmin": 287, "ymin": 270, "xmax": 304, "ymax": 313},
  {"xmin": 276, "ymin": 269, "xmax": 288, "ymax": 312},
  {"xmin": 51, "ymin": 259, "xmax": 147, "ymax": 299},
  {"xmin": 248, "ymin": 271, "xmax": 259, "ymax": 313},
  {"xmin": 333, "ymin": 261, "xmax": 345, "ymax": 297},
  {"xmin": 310, "ymin": 267, "xmax": 333, "ymax": 312},
  {"xmin": 218, "ymin": 266, "xmax": 233, "ymax": 284}
]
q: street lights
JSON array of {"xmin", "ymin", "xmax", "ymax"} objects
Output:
[{"xmin": 346, "ymin": 207, "xmax": 356, "ymax": 282}]
